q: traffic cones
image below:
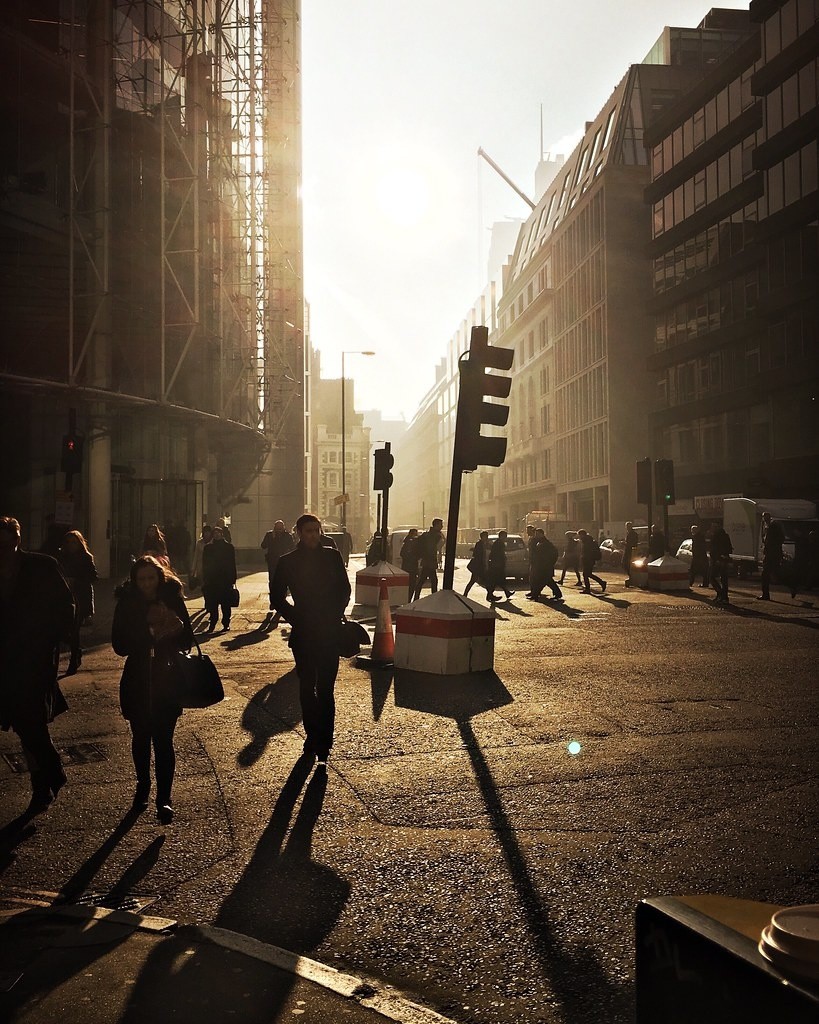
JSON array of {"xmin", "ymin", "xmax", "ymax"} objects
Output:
[{"xmin": 356, "ymin": 578, "xmax": 395, "ymax": 665}]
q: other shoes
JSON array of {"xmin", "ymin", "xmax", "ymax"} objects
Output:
[
  {"xmin": 574, "ymin": 582, "xmax": 582, "ymax": 586},
  {"xmin": 602, "ymin": 581, "xmax": 606, "ymax": 592},
  {"xmin": 756, "ymin": 595, "xmax": 770, "ymax": 601},
  {"xmin": 488, "ymin": 589, "xmax": 562, "ymax": 602},
  {"xmin": 157, "ymin": 803, "xmax": 176, "ymax": 817},
  {"xmin": 579, "ymin": 589, "xmax": 590, "ymax": 593},
  {"xmin": 224, "ymin": 625, "xmax": 230, "ymax": 630},
  {"xmin": 28, "ymin": 776, "xmax": 67, "ymax": 813},
  {"xmin": 65, "ymin": 655, "xmax": 82, "ymax": 677},
  {"xmin": 303, "ymin": 734, "xmax": 330, "ymax": 758},
  {"xmin": 555, "ymin": 579, "xmax": 563, "ymax": 585},
  {"xmin": 209, "ymin": 622, "xmax": 216, "ymax": 632},
  {"xmin": 792, "ymin": 582, "xmax": 801, "ymax": 598},
  {"xmin": 133, "ymin": 791, "xmax": 149, "ymax": 810},
  {"xmin": 714, "ymin": 589, "xmax": 729, "ymax": 604}
]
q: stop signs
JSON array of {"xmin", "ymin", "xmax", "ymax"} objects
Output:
[{"xmin": 374, "ymin": 531, "xmax": 382, "ymax": 541}]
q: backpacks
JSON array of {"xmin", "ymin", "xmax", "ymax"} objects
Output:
[
  {"xmin": 544, "ymin": 540, "xmax": 558, "ymax": 565},
  {"xmin": 406, "ymin": 532, "xmax": 430, "ymax": 561},
  {"xmin": 590, "ymin": 538, "xmax": 602, "ymax": 561}
]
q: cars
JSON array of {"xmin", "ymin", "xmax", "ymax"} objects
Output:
[
  {"xmin": 598, "ymin": 539, "xmax": 625, "ymax": 568},
  {"xmin": 676, "ymin": 538, "xmax": 711, "ymax": 572},
  {"xmin": 487, "ymin": 534, "xmax": 530, "ymax": 583}
]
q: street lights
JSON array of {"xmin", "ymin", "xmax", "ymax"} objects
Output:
[{"xmin": 340, "ymin": 351, "xmax": 376, "ymax": 525}]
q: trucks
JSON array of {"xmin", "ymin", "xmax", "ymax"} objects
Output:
[
  {"xmin": 526, "ymin": 510, "xmax": 598, "ymax": 570},
  {"xmin": 722, "ymin": 497, "xmax": 819, "ymax": 585}
]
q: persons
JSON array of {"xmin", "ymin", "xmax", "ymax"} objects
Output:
[
  {"xmin": 689, "ymin": 525, "xmax": 710, "ymax": 588},
  {"xmin": 270, "ymin": 515, "xmax": 351, "ymax": 749},
  {"xmin": 650, "ymin": 525, "xmax": 664, "ymax": 561},
  {"xmin": 202, "ymin": 527, "xmax": 237, "ymax": 631},
  {"xmin": 526, "ymin": 525, "xmax": 562, "ymax": 600},
  {"xmin": 62, "ymin": 530, "xmax": 99, "ymax": 676},
  {"xmin": 0, "ymin": 515, "xmax": 67, "ymax": 811},
  {"xmin": 413, "ymin": 519, "xmax": 443, "ymax": 602},
  {"xmin": 577, "ymin": 529, "xmax": 607, "ymax": 594},
  {"xmin": 192, "ymin": 526, "xmax": 214, "ymax": 613},
  {"xmin": 111, "ymin": 525, "xmax": 194, "ymax": 813},
  {"xmin": 557, "ymin": 531, "xmax": 582, "ymax": 586},
  {"xmin": 261, "ymin": 519, "xmax": 293, "ymax": 610},
  {"xmin": 707, "ymin": 521, "xmax": 732, "ymax": 605},
  {"xmin": 399, "ymin": 529, "xmax": 418, "ymax": 605},
  {"xmin": 464, "ymin": 531, "xmax": 502, "ymax": 603},
  {"xmin": 621, "ymin": 521, "xmax": 638, "ymax": 576},
  {"xmin": 342, "ymin": 527, "xmax": 353, "ymax": 568},
  {"xmin": 756, "ymin": 512, "xmax": 798, "ymax": 601},
  {"xmin": 489, "ymin": 531, "xmax": 516, "ymax": 600},
  {"xmin": 219, "ymin": 518, "xmax": 231, "ymax": 542}
]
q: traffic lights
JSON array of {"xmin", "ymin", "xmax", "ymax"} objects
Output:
[
  {"xmin": 457, "ymin": 324, "xmax": 516, "ymax": 467},
  {"xmin": 654, "ymin": 459, "xmax": 676, "ymax": 506},
  {"xmin": 373, "ymin": 449, "xmax": 394, "ymax": 490},
  {"xmin": 61, "ymin": 435, "xmax": 82, "ymax": 471}
]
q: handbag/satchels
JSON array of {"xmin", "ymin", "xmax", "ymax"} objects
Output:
[
  {"xmin": 337, "ymin": 614, "xmax": 371, "ymax": 658},
  {"xmin": 179, "ymin": 630, "xmax": 224, "ymax": 708},
  {"xmin": 468, "ymin": 558, "xmax": 475, "ymax": 573},
  {"xmin": 230, "ymin": 582, "xmax": 239, "ymax": 607}
]
